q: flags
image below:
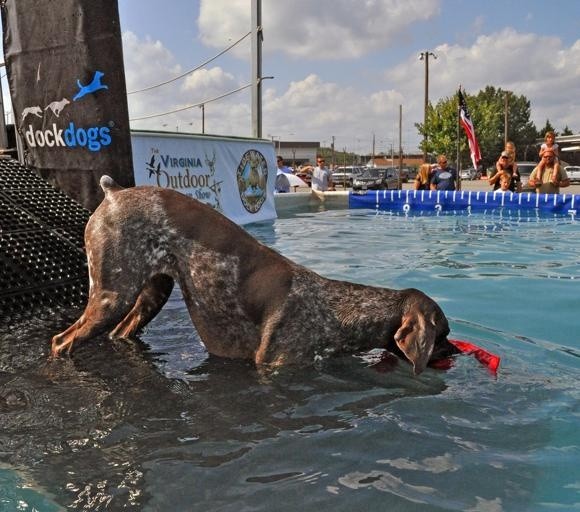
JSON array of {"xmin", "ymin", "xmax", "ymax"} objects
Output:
[{"xmin": 458, "ymin": 89, "xmax": 482, "ymax": 172}]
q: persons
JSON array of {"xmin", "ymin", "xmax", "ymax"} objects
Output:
[
  {"xmin": 275, "ymin": 156, "xmax": 290, "ymax": 192},
  {"xmin": 534, "ymin": 131, "xmax": 560, "ymax": 186},
  {"xmin": 415, "ymin": 155, "xmax": 462, "ymax": 191},
  {"xmin": 528, "ymin": 150, "xmax": 570, "ymax": 194},
  {"xmin": 493, "ymin": 173, "xmax": 512, "ymax": 192},
  {"xmin": 300, "ymin": 155, "xmax": 334, "ymax": 191},
  {"xmin": 496, "ymin": 141, "xmax": 519, "ymax": 177},
  {"xmin": 488, "ymin": 156, "xmax": 521, "ymax": 192}
]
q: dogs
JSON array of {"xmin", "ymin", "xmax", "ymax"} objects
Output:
[{"xmin": 50, "ymin": 173, "xmax": 463, "ymax": 376}]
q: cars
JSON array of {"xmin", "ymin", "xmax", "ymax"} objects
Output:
[
  {"xmin": 460, "ymin": 168, "xmax": 481, "ymax": 180},
  {"xmin": 288, "ymin": 165, "xmax": 418, "ymax": 190},
  {"xmin": 564, "ymin": 166, "xmax": 580, "ymax": 182},
  {"xmin": 514, "ymin": 163, "xmax": 538, "ymax": 191}
]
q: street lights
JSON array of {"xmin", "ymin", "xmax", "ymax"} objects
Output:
[{"xmin": 419, "ymin": 51, "xmax": 436, "ymax": 164}]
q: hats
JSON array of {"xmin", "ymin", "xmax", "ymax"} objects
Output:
[{"xmin": 501, "ymin": 151, "xmax": 510, "ymax": 159}]
